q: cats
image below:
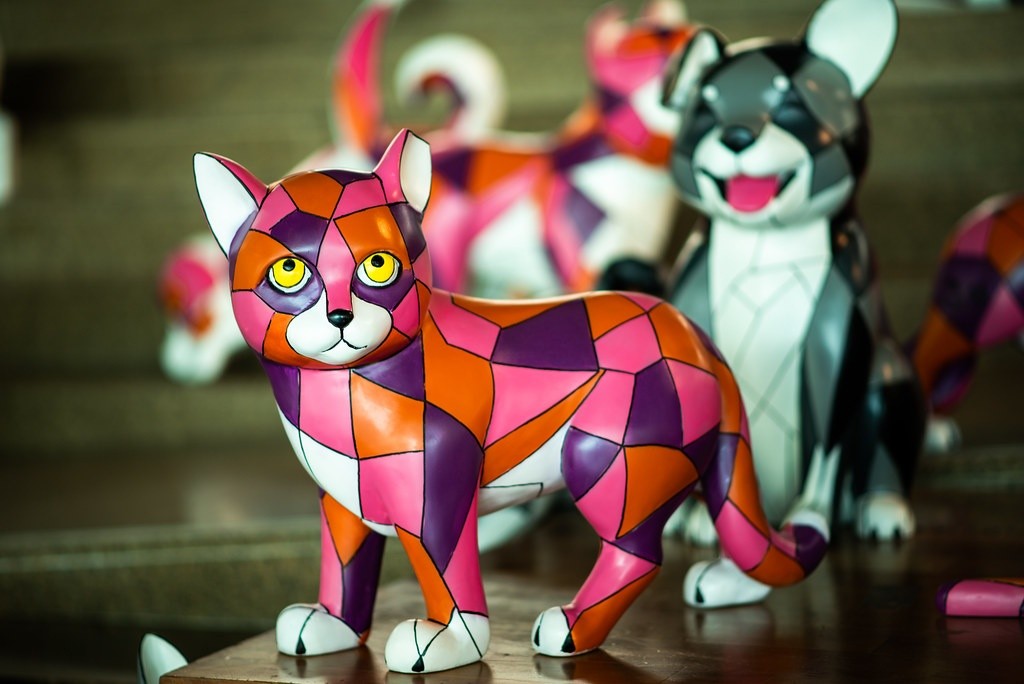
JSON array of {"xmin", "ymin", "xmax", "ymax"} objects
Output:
[
  {"xmin": 193, "ymin": 127, "xmax": 832, "ymax": 674},
  {"xmin": 158, "ymin": 0, "xmax": 1024, "ymax": 462}
]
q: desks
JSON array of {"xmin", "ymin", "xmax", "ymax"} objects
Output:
[{"xmin": 158, "ymin": 491, "xmax": 1024, "ymax": 684}]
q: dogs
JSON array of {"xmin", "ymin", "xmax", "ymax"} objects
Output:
[{"xmin": 653, "ymin": 0, "xmax": 929, "ymax": 549}]
